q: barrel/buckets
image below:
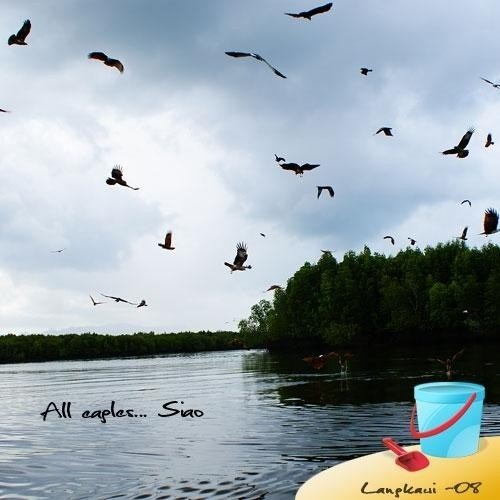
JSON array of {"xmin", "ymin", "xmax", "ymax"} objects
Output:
[{"xmin": 411, "ymin": 382, "xmax": 486, "ymax": 457}]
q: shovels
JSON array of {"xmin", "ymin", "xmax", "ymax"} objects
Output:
[{"xmin": 382, "ymin": 436, "xmax": 430, "ymax": 472}]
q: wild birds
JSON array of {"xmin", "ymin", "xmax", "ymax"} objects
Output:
[
  {"xmin": 105, "ymin": 164, "xmax": 140, "ymax": 191},
  {"xmin": 225, "ymin": 50, "xmax": 286, "ymax": 78},
  {"xmin": 87, "ymin": 51, "xmax": 124, "ymax": 74},
  {"xmin": 88, "ymin": 292, "xmax": 148, "ymax": 308},
  {"xmin": 375, "ymin": 126, "xmax": 395, "ymax": 137},
  {"xmin": 482, "ymin": 77, "xmax": 499, "ymax": 90},
  {"xmin": 283, "ymin": 2, "xmax": 333, "ymax": 20},
  {"xmin": 315, "ymin": 184, "xmax": 334, "ymax": 198},
  {"xmin": 272, "ymin": 153, "xmax": 321, "ymax": 177},
  {"xmin": 383, "ymin": 235, "xmax": 394, "ymax": 245},
  {"xmin": 7, "ymin": 19, "xmax": 31, "ymax": 46},
  {"xmin": 267, "ymin": 285, "xmax": 284, "ymax": 291},
  {"xmin": 452, "ymin": 200, "xmax": 499, "ymax": 241},
  {"xmin": 224, "ymin": 242, "xmax": 253, "ymax": 274},
  {"xmin": 360, "ymin": 68, "xmax": 373, "ymax": 76},
  {"xmin": 484, "ymin": 133, "xmax": 494, "ymax": 148},
  {"xmin": 442, "ymin": 128, "xmax": 475, "ymax": 159},
  {"xmin": 408, "ymin": 237, "xmax": 416, "ymax": 246},
  {"xmin": 158, "ymin": 232, "xmax": 175, "ymax": 251}
]
